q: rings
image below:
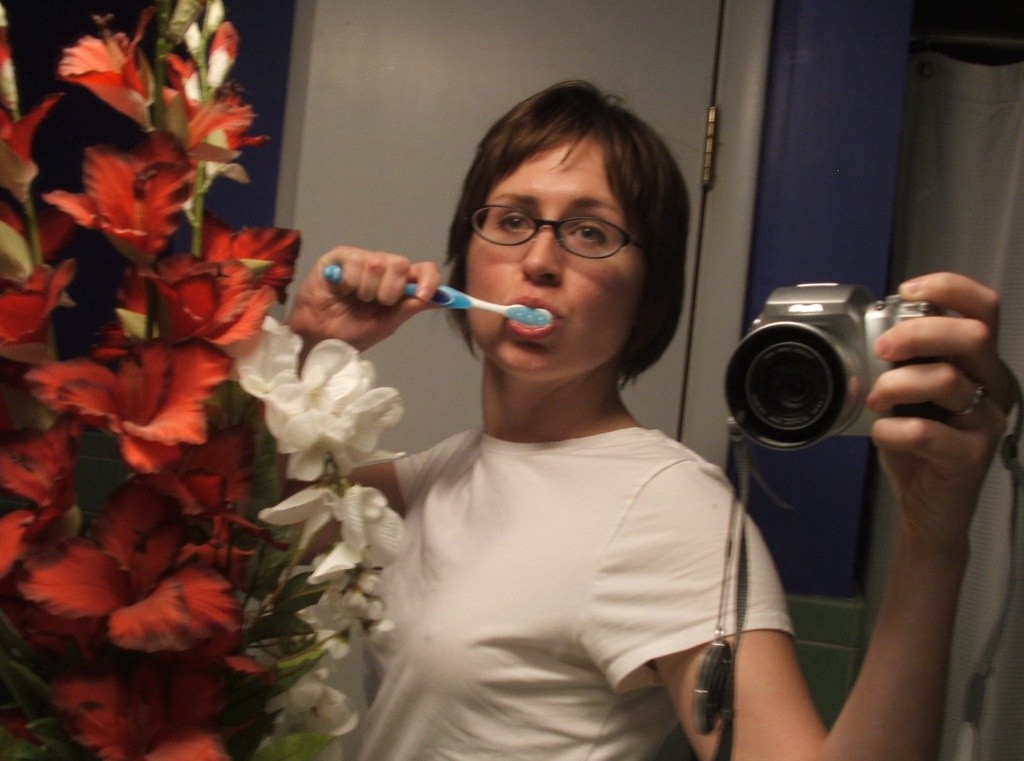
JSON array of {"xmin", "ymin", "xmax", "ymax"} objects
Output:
[{"xmin": 952, "ymin": 382, "xmax": 985, "ymax": 416}]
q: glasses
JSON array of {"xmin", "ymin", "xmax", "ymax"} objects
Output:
[{"xmin": 472, "ymin": 205, "xmax": 645, "ymax": 259}]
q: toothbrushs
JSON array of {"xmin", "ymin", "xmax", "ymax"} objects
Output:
[{"xmin": 320, "ymin": 263, "xmax": 554, "ymax": 329}]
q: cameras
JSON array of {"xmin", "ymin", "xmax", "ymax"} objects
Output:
[{"xmin": 724, "ymin": 282, "xmax": 946, "ymax": 453}]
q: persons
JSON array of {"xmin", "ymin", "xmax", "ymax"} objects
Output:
[{"xmin": 258, "ymin": 77, "xmax": 1017, "ymax": 761}]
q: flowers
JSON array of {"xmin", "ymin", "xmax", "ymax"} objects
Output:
[{"xmin": 0, "ymin": 0, "xmax": 407, "ymax": 760}]
